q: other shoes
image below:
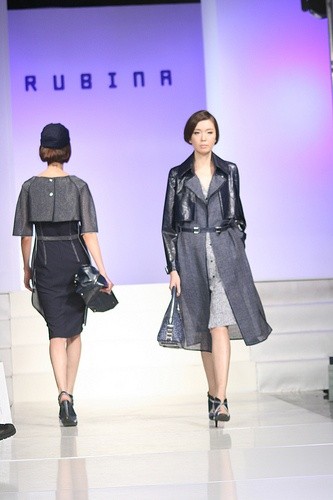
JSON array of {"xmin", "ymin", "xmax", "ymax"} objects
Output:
[{"xmin": 0, "ymin": 423, "xmax": 16, "ymax": 441}]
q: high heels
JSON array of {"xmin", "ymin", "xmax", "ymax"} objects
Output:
[
  {"xmin": 208, "ymin": 392, "xmax": 230, "ymax": 428},
  {"xmin": 58, "ymin": 391, "xmax": 78, "ymax": 429}
]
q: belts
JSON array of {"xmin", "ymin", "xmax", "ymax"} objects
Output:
[{"xmin": 178, "ymin": 225, "xmax": 230, "ymax": 233}]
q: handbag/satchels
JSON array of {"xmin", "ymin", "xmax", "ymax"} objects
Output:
[
  {"xmin": 73, "ymin": 265, "xmax": 120, "ymax": 326},
  {"xmin": 157, "ymin": 286, "xmax": 186, "ymax": 349}
]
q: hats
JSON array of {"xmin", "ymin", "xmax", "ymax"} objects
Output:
[{"xmin": 40, "ymin": 123, "xmax": 70, "ymax": 149}]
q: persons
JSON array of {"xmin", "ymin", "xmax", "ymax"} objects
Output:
[
  {"xmin": 12, "ymin": 123, "xmax": 115, "ymax": 428},
  {"xmin": 161, "ymin": 110, "xmax": 273, "ymax": 428}
]
